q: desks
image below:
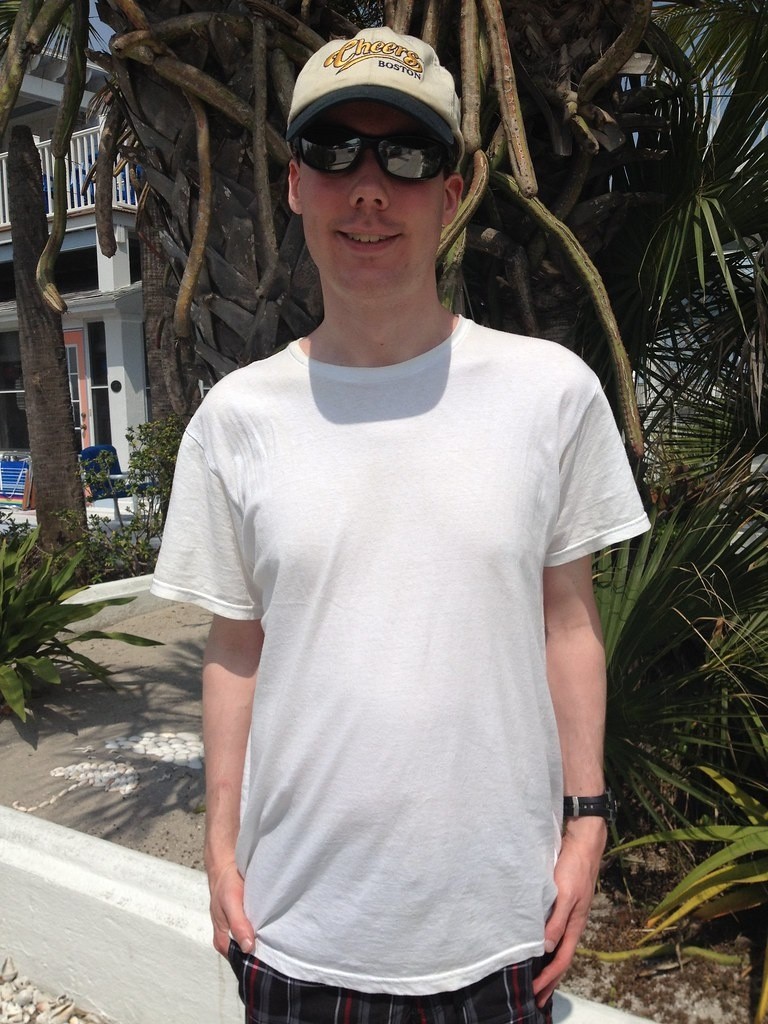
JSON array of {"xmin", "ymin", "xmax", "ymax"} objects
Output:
[{"xmin": 107, "ymin": 473, "xmax": 130, "ymax": 480}]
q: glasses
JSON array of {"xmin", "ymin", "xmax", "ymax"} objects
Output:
[{"xmin": 292, "ymin": 121, "xmax": 448, "ymax": 181}]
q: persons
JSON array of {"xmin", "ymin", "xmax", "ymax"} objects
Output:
[{"xmin": 151, "ymin": 25, "xmax": 652, "ymax": 1024}]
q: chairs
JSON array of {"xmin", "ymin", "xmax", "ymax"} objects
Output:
[
  {"xmin": 0, "ymin": 453, "xmax": 33, "ymax": 511},
  {"xmin": 82, "ymin": 445, "xmax": 158, "ymax": 500}
]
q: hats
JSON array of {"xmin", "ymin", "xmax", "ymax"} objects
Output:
[{"xmin": 286, "ymin": 27, "xmax": 465, "ymax": 175}]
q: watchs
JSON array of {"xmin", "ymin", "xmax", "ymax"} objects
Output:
[{"xmin": 563, "ymin": 787, "xmax": 617, "ymax": 828}]
